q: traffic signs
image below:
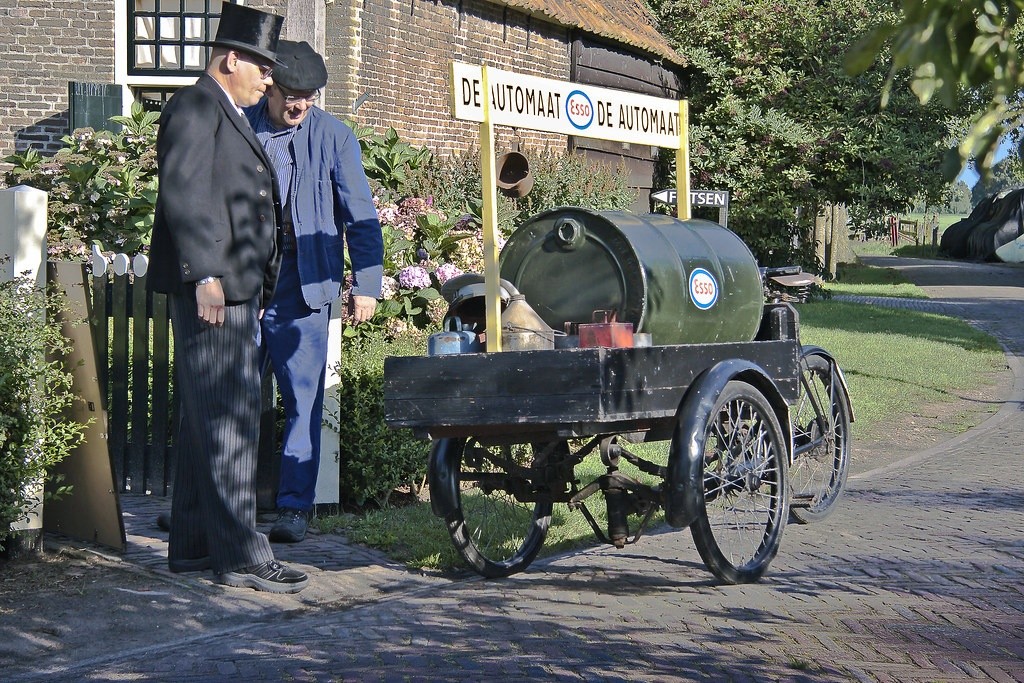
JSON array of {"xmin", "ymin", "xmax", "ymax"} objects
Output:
[{"xmin": 650, "ymin": 186, "xmax": 727, "ymax": 206}]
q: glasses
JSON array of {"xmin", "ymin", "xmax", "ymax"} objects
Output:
[
  {"xmin": 238, "ymin": 50, "xmax": 273, "ymax": 80},
  {"xmin": 273, "ymin": 80, "xmax": 321, "ymax": 104}
]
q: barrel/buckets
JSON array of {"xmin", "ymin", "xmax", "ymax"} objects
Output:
[
  {"xmin": 499, "ymin": 206, "xmax": 764, "ymax": 346},
  {"xmin": 501, "ymin": 295, "xmax": 554, "ymax": 351}
]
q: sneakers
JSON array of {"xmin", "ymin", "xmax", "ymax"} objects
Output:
[
  {"xmin": 214, "ymin": 559, "xmax": 309, "ymax": 594},
  {"xmin": 169, "ymin": 557, "xmax": 212, "ymax": 573},
  {"xmin": 269, "ymin": 507, "xmax": 310, "ymax": 544}
]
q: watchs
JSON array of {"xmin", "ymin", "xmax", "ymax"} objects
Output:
[{"xmin": 194, "ymin": 276, "xmax": 219, "ymax": 287}]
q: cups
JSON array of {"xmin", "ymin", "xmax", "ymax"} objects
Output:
[
  {"xmin": 554, "ymin": 335, "xmax": 579, "ymax": 349},
  {"xmin": 633, "ymin": 333, "xmax": 652, "ymax": 347}
]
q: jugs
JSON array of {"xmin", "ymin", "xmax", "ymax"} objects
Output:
[{"xmin": 495, "ymin": 141, "xmax": 534, "ymax": 198}]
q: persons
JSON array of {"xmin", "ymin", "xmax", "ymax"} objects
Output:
[
  {"xmin": 143, "ymin": 2, "xmax": 307, "ymax": 593},
  {"xmin": 156, "ymin": 41, "xmax": 384, "ymax": 542}
]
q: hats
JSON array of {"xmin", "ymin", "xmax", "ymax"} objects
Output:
[
  {"xmin": 199, "ymin": 1, "xmax": 288, "ymax": 68},
  {"xmin": 269, "ymin": 39, "xmax": 328, "ymax": 91}
]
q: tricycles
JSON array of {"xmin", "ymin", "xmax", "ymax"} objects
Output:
[{"xmin": 381, "ymin": 263, "xmax": 858, "ymax": 586}]
